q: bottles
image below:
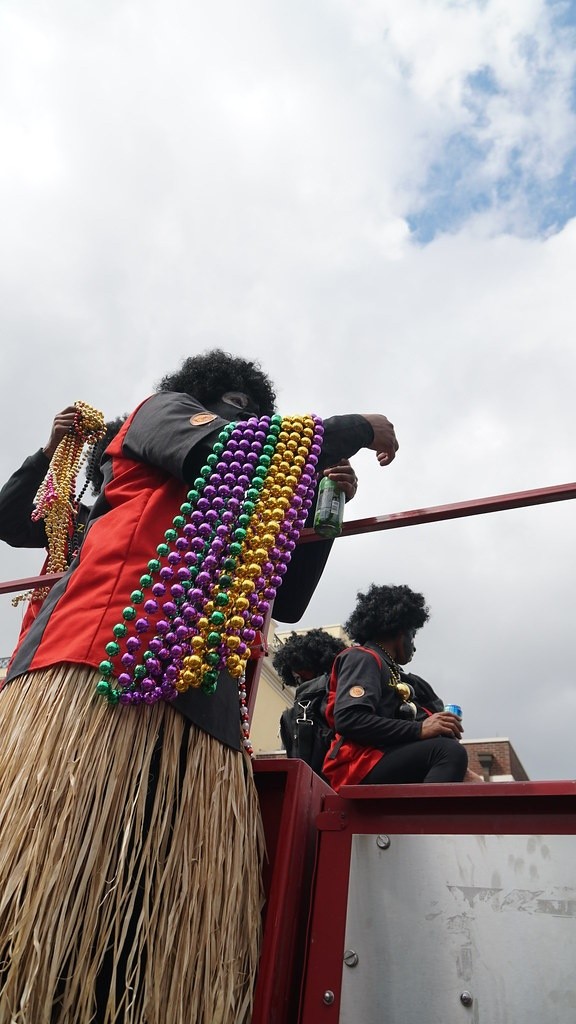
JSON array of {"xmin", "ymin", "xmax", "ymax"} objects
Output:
[{"xmin": 313, "ymin": 469, "xmax": 346, "ymax": 538}]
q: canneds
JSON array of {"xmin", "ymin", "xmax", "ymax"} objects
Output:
[{"xmin": 442, "ymin": 704, "xmax": 462, "ymax": 724}]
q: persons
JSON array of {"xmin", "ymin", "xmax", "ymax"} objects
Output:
[
  {"xmin": 0, "ymin": 406, "xmax": 128, "ymax": 687},
  {"xmin": 276, "ymin": 628, "xmax": 347, "ymax": 785},
  {"xmin": 0, "ymin": 350, "xmax": 398, "ymax": 1024},
  {"xmin": 321, "ymin": 584, "xmax": 467, "ymax": 792}
]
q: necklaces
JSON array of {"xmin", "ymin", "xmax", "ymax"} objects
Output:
[
  {"xmin": 95, "ymin": 413, "xmax": 323, "ymax": 756},
  {"xmin": 10, "ymin": 402, "xmax": 107, "ymax": 606},
  {"xmin": 374, "ymin": 642, "xmax": 399, "ymax": 680}
]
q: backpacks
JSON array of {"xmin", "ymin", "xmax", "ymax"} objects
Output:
[
  {"xmin": 279, "ymin": 672, "xmax": 334, "ymax": 784},
  {"xmin": 397, "ymin": 670, "xmax": 445, "ymax": 720}
]
